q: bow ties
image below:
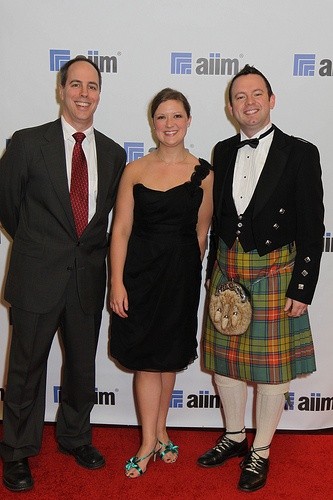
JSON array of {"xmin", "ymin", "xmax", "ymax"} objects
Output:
[{"xmin": 237, "ymin": 138, "xmax": 259, "ymax": 150}]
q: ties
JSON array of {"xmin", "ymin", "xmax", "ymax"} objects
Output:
[{"xmin": 69, "ymin": 132, "xmax": 89, "ymax": 238}]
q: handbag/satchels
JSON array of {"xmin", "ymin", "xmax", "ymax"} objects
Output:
[{"xmin": 209, "ymin": 254, "xmax": 295, "ymax": 335}]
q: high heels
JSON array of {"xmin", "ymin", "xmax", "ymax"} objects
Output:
[
  {"xmin": 157, "ymin": 440, "xmax": 179, "ymax": 464},
  {"xmin": 125, "ymin": 439, "xmax": 159, "ymax": 479}
]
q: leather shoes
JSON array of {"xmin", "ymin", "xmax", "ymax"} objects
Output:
[
  {"xmin": 2, "ymin": 458, "xmax": 34, "ymax": 491},
  {"xmin": 57, "ymin": 442, "xmax": 105, "ymax": 469},
  {"xmin": 238, "ymin": 444, "xmax": 272, "ymax": 492},
  {"xmin": 197, "ymin": 428, "xmax": 248, "ymax": 467}
]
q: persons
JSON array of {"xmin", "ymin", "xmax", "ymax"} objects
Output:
[
  {"xmin": 1, "ymin": 59, "xmax": 127, "ymax": 493},
  {"xmin": 201, "ymin": 64, "xmax": 325, "ymax": 492},
  {"xmin": 109, "ymin": 88, "xmax": 214, "ymax": 478}
]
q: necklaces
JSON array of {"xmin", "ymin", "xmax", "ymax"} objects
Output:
[{"xmin": 156, "ymin": 147, "xmax": 189, "ymax": 165}]
